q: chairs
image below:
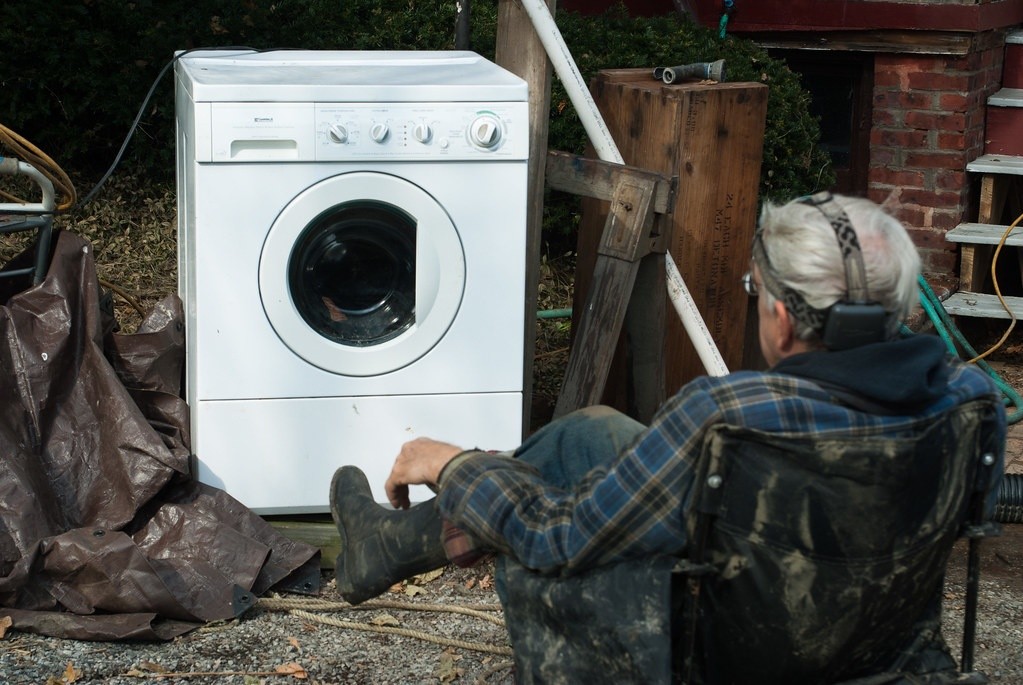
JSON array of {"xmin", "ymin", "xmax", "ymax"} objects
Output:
[{"xmin": 491, "ymin": 393, "xmax": 1002, "ymax": 685}]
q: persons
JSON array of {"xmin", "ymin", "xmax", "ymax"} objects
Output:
[{"xmin": 330, "ymin": 193, "xmax": 1007, "ymax": 683}]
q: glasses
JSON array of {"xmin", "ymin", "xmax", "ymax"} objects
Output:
[{"xmin": 742, "ymin": 272, "xmax": 766, "ymax": 295}]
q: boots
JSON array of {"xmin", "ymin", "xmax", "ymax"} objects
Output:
[{"xmin": 329, "ymin": 465, "xmax": 453, "ymax": 606}]
